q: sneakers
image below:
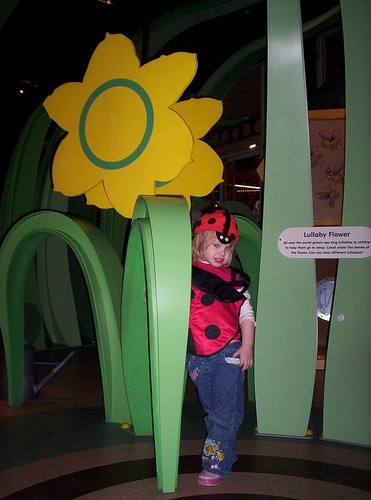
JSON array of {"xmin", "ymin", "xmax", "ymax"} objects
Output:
[{"xmin": 196, "ymin": 470, "xmax": 222, "ymax": 486}]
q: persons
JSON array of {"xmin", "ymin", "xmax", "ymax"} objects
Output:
[{"xmin": 187, "ymin": 205, "xmax": 255, "ymax": 485}]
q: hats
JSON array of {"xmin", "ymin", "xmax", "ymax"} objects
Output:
[{"xmin": 194, "ymin": 204, "xmax": 238, "ymax": 245}]
